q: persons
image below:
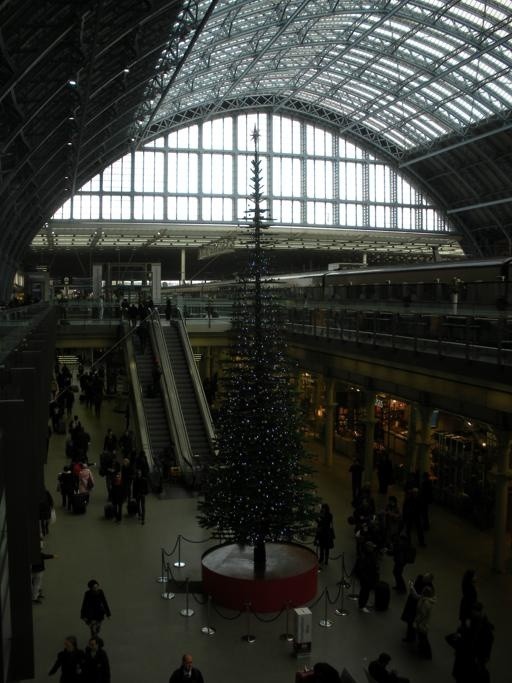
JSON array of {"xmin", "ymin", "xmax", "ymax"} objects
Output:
[
  {"xmin": 85, "ymin": 636, "xmax": 110, "ymax": 683},
  {"xmin": 48, "ymin": 355, "xmax": 74, "ymax": 436},
  {"xmin": 121, "ymin": 296, "xmax": 155, "ymax": 327},
  {"xmin": 165, "ymin": 296, "xmax": 172, "ymax": 321},
  {"xmin": 347, "ymin": 420, "xmax": 496, "ymax": 683},
  {"xmin": 99, "ymin": 429, "xmax": 177, "ymax": 525},
  {"xmin": 80, "ymin": 580, "xmax": 111, "ymax": 636},
  {"xmin": 52, "ymin": 294, "xmax": 71, "ymax": 326},
  {"xmin": 76, "ymin": 363, "xmax": 120, "ymax": 419},
  {"xmin": 48, "ymin": 635, "xmax": 86, "ymax": 683},
  {"xmin": 149, "ymin": 363, "xmax": 163, "ymax": 392},
  {"xmin": 314, "ymin": 504, "xmax": 333, "ymax": 565},
  {"xmin": 133, "ymin": 321, "xmax": 150, "ymax": 356},
  {"xmin": 79, "ymin": 295, "xmax": 104, "ymax": 320},
  {"xmin": 31, "ymin": 536, "xmax": 58, "ymax": 606},
  {"xmin": 38, "ymin": 484, "xmax": 54, "ymax": 538},
  {"xmin": 57, "ymin": 417, "xmax": 94, "ymax": 511},
  {"xmin": 124, "ymin": 405, "xmax": 130, "ymax": 431},
  {"xmin": 208, "ymin": 295, "xmax": 215, "ymax": 319},
  {"xmin": 301, "ymin": 662, "xmax": 344, "ymax": 683},
  {"xmin": 169, "ymin": 654, "xmax": 205, "ymax": 683}
]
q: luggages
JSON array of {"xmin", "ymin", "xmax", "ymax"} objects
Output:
[{"xmin": 73, "ymin": 490, "xmax": 86, "ymax": 516}]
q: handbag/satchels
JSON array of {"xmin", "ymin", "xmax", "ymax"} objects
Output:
[
  {"xmin": 330, "ymin": 526, "xmax": 336, "ymax": 540},
  {"xmin": 87, "ymin": 473, "xmax": 94, "ymax": 489}
]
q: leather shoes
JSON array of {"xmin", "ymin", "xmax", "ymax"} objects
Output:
[{"xmin": 392, "ymin": 585, "xmax": 408, "ymax": 595}]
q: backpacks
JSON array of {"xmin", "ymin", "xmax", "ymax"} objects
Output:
[{"xmin": 406, "ymin": 544, "xmax": 416, "ymax": 563}]
